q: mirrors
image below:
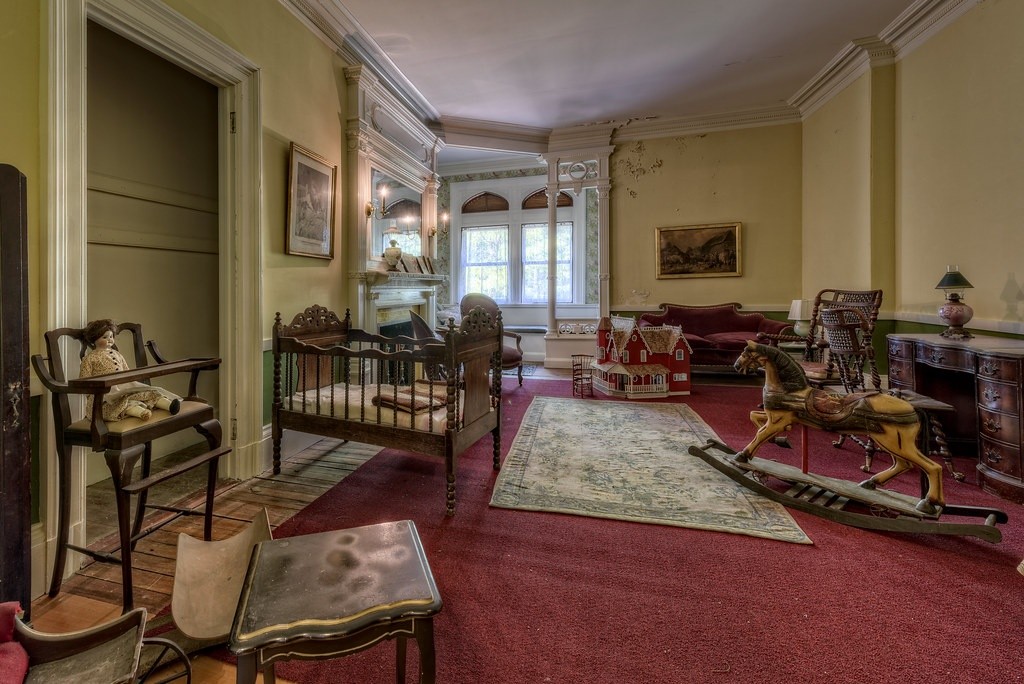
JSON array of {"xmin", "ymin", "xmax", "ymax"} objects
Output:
[{"xmin": 370, "ymin": 167, "xmax": 423, "ymax": 259}]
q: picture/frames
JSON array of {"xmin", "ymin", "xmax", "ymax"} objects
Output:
[
  {"xmin": 654, "ymin": 222, "xmax": 743, "ymax": 280},
  {"xmin": 285, "ymin": 141, "xmax": 337, "ymax": 260}
]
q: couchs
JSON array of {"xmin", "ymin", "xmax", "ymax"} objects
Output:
[{"xmin": 638, "ymin": 302, "xmax": 794, "ymax": 377}]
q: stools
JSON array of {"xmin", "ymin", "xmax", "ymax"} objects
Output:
[{"xmin": 222, "ymin": 520, "xmax": 444, "ymax": 684}]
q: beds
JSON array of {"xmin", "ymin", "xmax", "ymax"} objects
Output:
[{"xmin": 269, "ymin": 304, "xmax": 505, "ymax": 514}]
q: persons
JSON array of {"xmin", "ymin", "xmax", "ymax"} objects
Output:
[{"xmin": 79, "ymin": 319, "xmax": 183, "ymax": 421}]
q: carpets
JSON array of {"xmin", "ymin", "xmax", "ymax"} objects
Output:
[{"xmin": 486, "ymin": 398, "xmax": 815, "ymax": 547}]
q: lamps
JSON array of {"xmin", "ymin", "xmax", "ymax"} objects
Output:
[
  {"xmin": 436, "ymin": 212, "xmax": 448, "ymax": 244},
  {"xmin": 383, "ymin": 226, "xmax": 404, "ymax": 273},
  {"xmin": 789, "ymin": 298, "xmax": 818, "ymax": 343},
  {"xmin": 406, "ymin": 216, "xmax": 421, "ymax": 240},
  {"xmin": 935, "ymin": 265, "xmax": 976, "ymax": 339},
  {"xmin": 365, "ymin": 185, "xmax": 389, "ymax": 219}
]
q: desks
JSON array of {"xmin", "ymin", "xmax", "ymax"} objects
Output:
[
  {"xmin": 780, "ymin": 343, "xmax": 818, "ymax": 363},
  {"xmin": 888, "ymin": 332, "xmax": 1024, "ymax": 506}
]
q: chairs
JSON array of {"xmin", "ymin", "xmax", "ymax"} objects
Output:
[
  {"xmin": 30, "ymin": 322, "xmax": 232, "ymax": 615},
  {"xmin": 571, "ymin": 354, "xmax": 595, "ymax": 393},
  {"xmin": 571, "ymin": 359, "xmax": 593, "ymax": 398},
  {"xmin": 756, "ymin": 287, "xmax": 884, "ymax": 390},
  {"xmin": 461, "ymin": 293, "xmax": 525, "ymax": 387},
  {"xmin": 819, "ymin": 306, "xmax": 966, "ymax": 483}
]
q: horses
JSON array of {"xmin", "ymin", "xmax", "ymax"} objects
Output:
[{"xmin": 734, "ymin": 339, "xmax": 944, "ymax": 514}]
